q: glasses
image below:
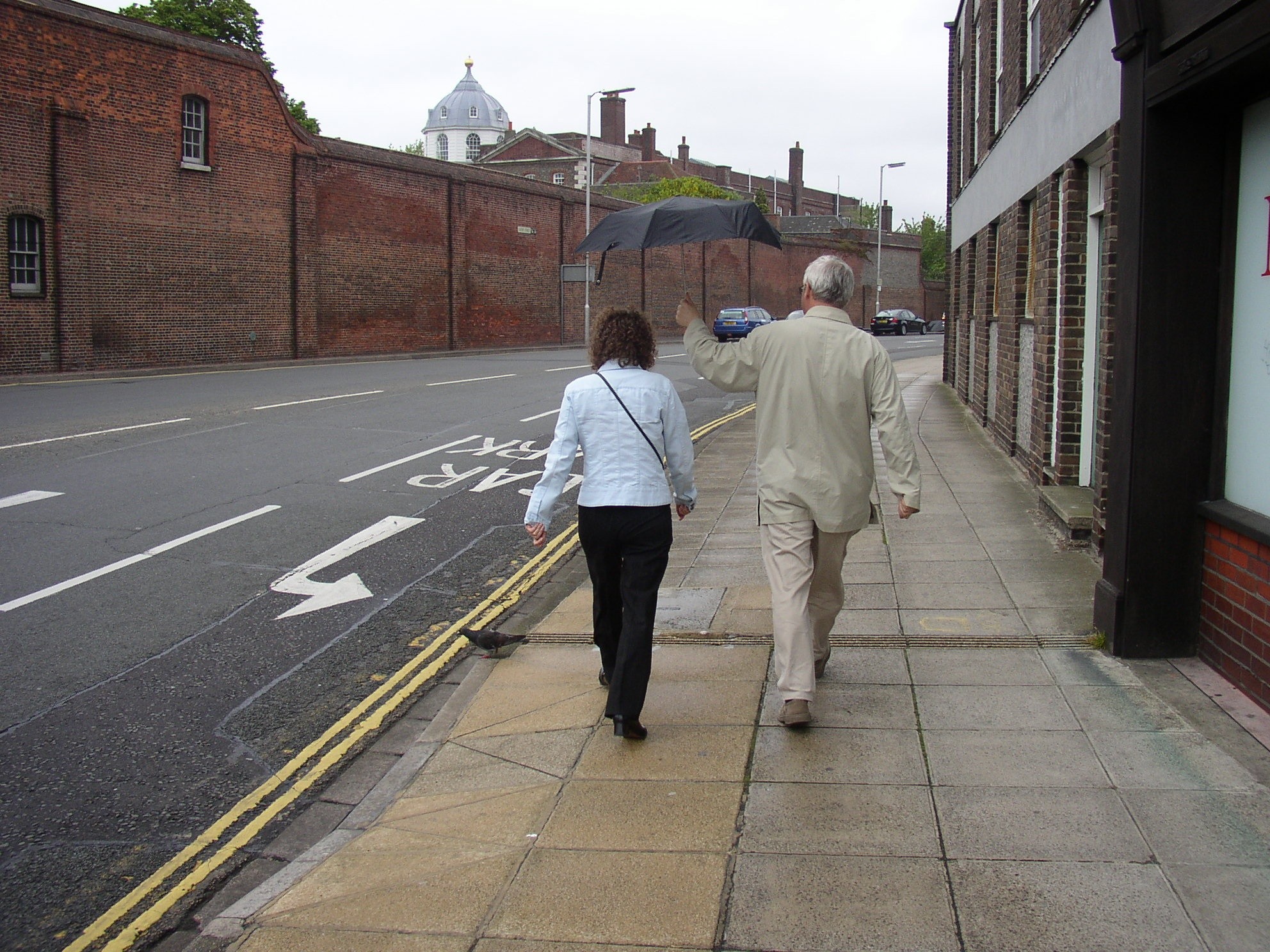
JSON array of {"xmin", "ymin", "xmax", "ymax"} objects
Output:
[{"xmin": 799, "ymin": 284, "xmax": 811, "ymax": 294}]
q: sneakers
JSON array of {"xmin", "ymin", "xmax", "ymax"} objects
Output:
[
  {"xmin": 778, "ymin": 699, "xmax": 811, "ymax": 725},
  {"xmin": 815, "ymin": 642, "xmax": 831, "ymax": 678}
]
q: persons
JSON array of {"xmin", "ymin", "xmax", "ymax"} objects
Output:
[
  {"xmin": 525, "ymin": 301, "xmax": 698, "ymax": 739},
  {"xmin": 677, "ymin": 255, "xmax": 921, "ymax": 724}
]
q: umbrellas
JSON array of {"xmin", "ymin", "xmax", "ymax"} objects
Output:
[{"xmin": 571, "ymin": 194, "xmax": 783, "ymax": 300}]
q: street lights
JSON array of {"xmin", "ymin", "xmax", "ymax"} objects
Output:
[
  {"xmin": 585, "ymin": 87, "xmax": 636, "ymax": 348},
  {"xmin": 875, "ymin": 162, "xmax": 906, "ymax": 316}
]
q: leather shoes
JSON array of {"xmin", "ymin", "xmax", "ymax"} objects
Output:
[
  {"xmin": 599, "ymin": 666, "xmax": 608, "ymax": 685},
  {"xmin": 613, "ymin": 714, "xmax": 647, "ymax": 739}
]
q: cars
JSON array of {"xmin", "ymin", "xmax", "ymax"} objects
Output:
[{"xmin": 870, "ymin": 309, "xmax": 927, "ymax": 336}]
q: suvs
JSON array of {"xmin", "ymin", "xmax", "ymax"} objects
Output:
[{"xmin": 712, "ymin": 306, "xmax": 776, "ymax": 343}]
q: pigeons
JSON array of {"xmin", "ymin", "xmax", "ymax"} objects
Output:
[{"xmin": 456, "ymin": 627, "xmax": 527, "ymax": 659}]
